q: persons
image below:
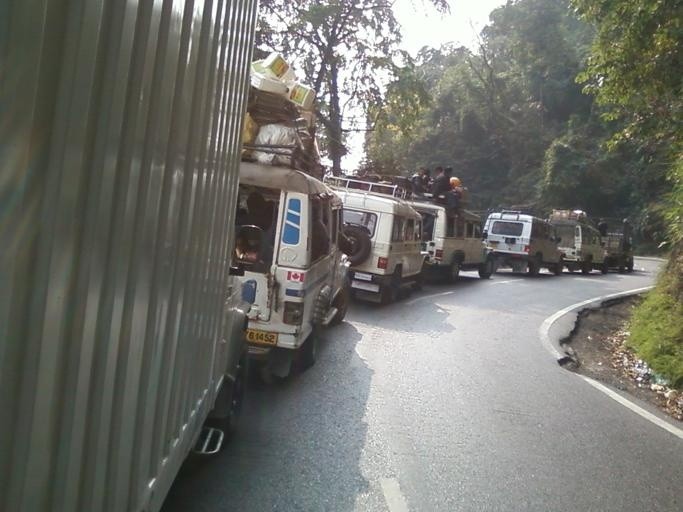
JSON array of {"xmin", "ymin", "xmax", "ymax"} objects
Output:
[
  {"xmin": 413, "ymin": 165, "xmax": 459, "ymax": 217},
  {"xmin": 598, "ymin": 218, "xmax": 608, "ymax": 236}
]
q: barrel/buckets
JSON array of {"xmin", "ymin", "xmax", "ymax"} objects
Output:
[
  {"xmin": 240, "ymin": 270, "xmax": 268, "ymax": 308},
  {"xmin": 250, "ymin": 51, "xmax": 317, "ymax": 110}
]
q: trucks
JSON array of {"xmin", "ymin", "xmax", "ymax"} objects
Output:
[{"xmin": 0, "ymin": 0, "xmax": 267, "ymax": 511}]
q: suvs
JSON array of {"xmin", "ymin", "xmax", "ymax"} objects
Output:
[
  {"xmin": 320, "ymin": 172, "xmax": 430, "ymax": 304},
  {"xmin": 596, "ymin": 213, "xmax": 638, "ymax": 275},
  {"xmin": 408, "ymin": 191, "xmax": 495, "ymax": 283},
  {"xmin": 541, "ymin": 209, "xmax": 609, "ymax": 274},
  {"xmin": 228, "ymin": 135, "xmax": 358, "ymax": 386},
  {"xmin": 479, "ymin": 208, "xmax": 566, "ymax": 278}
]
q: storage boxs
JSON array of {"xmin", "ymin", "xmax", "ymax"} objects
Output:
[
  {"xmin": 253, "ymin": 74, "xmax": 285, "ymax": 94},
  {"xmin": 249, "ymin": 59, "xmax": 266, "ymax": 74},
  {"xmin": 261, "ymin": 53, "xmax": 287, "ymax": 80},
  {"xmin": 287, "ymin": 78, "xmax": 316, "ymax": 109}
]
